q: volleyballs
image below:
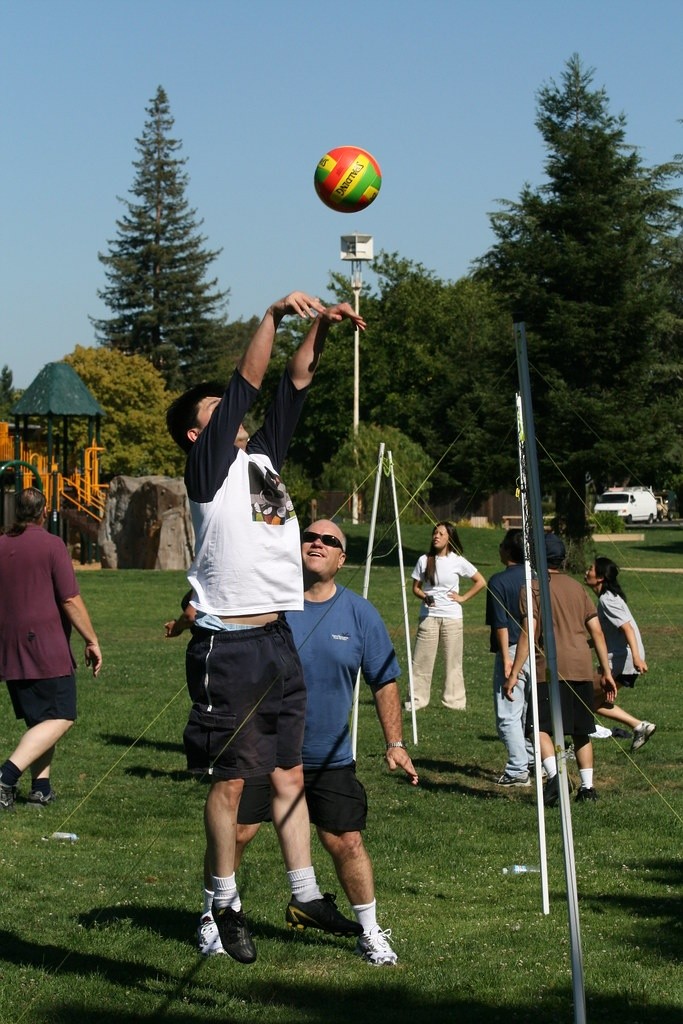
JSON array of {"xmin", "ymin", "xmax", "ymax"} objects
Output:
[{"xmin": 314, "ymin": 146, "xmax": 382, "ymax": 213}]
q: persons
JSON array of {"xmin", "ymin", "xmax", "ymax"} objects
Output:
[
  {"xmin": 162, "ymin": 294, "xmax": 367, "ymax": 966},
  {"xmin": 0, "ymin": 488, "xmax": 103, "ymax": 809},
  {"xmin": 164, "ymin": 518, "xmax": 418, "ymax": 966},
  {"xmin": 404, "ymin": 519, "xmax": 488, "ymax": 712},
  {"xmin": 564, "ymin": 556, "xmax": 657, "ymax": 760},
  {"xmin": 487, "ymin": 529, "xmax": 619, "ymax": 808}
]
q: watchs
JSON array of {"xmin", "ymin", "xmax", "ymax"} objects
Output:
[{"xmin": 387, "ymin": 741, "xmax": 408, "ymax": 749}]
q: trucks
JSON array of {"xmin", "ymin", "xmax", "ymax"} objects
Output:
[{"xmin": 654, "ymin": 494, "xmax": 674, "ymax": 521}]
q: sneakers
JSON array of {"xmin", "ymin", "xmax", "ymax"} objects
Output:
[
  {"xmin": 575, "ymin": 787, "xmax": 597, "ymax": 803},
  {"xmin": 528, "ymin": 763, "xmax": 548, "ymax": 777},
  {"xmin": 286, "ymin": 892, "xmax": 364, "ymax": 937},
  {"xmin": 543, "ymin": 774, "xmax": 573, "ymax": 807},
  {"xmin": 631, "ymin": 720, "xmax": 657, "ymax": 751},
  {"xmin": 194, "ymin": 910, "xmax": 227, "ymax": 957},
  {"xmin": 0, "ymin": 770, "xmax": 19, "ymax": 810},
  {"xmin": 27, "ymin": 785, "xmax": 56, "ymax": 807},
  {"xmin": 493, "ymin": 772, "xmax": 532, "ymax": 788},
  {"xmin": 211, "ymin": 898, "xmax": 257, "ymax": 964},
  {"xmin": 357, "ymin": 925, "xmax": 398, "ymax": 967}
]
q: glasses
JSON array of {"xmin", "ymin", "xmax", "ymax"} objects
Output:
[{"xmin": 301, "ymin": 531, "xmax": 343, "ymax": 552}]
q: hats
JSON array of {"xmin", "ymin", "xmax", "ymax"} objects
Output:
[{"xmin": 545, "ymin": 532, "xmax": 565, "ymax": 568}]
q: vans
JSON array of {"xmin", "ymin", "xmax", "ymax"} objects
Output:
[{"xmin": 594, "ymin": 486, "xmax": 658, "ymax": 525}]
